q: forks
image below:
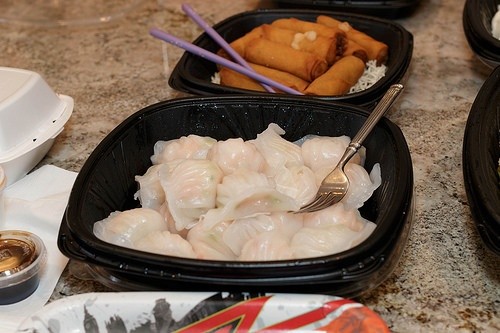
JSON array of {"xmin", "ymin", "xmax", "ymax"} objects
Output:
[{"xmin": 286, "ymin": 84, "xmax": 405, "ymax": 213}]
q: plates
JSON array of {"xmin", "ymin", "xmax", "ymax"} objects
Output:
[{"xmin": 18, "ymin": 292, "xmax": 390, "ymax": 333}]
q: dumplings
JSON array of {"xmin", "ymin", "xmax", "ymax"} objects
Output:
[{"xmin": 92, "ymin": 122, "xmax": 381, "ymax": 260}]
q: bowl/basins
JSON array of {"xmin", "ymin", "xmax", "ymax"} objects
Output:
[
  {"xmin": 462, "ymin": 0, "xmax": 500, "ymax": 69},
  {"xmin": 278, "ymin": 0, "xmax": 421, "ymax": 18},
  {"xmin": 57, "ymin": 91, "xmax": 415, "ymax": 299},
  {"xmin": 0, "ymin": 230, "xmax": 47, "ymax": 306},
  {"xmin": 462, "ymin": 65, "xmax": 500, "ymax": 256},
  {"xmin": 168, "ymin": 8, "xmax": 414, "ymax": 109}
]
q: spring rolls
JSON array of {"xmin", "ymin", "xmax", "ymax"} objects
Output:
[{"xmin": 215, "ymin": 16, "xmax": 389, "ymax": 95}]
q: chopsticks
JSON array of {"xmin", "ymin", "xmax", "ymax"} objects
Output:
[{"xmin": 149, "ymin": 5, "xmax": 304, "ymax": 94}]
